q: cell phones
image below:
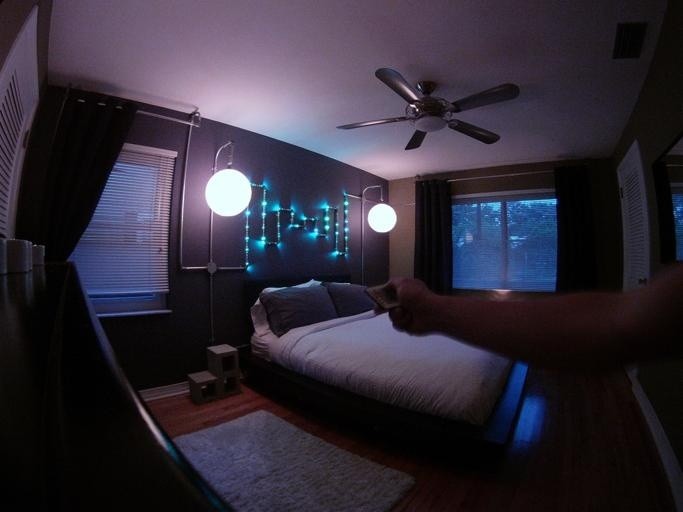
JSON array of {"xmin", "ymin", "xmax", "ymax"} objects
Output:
[{"xmin": 364, "ymin": 284, "xmax": 402, "ymax": 311}]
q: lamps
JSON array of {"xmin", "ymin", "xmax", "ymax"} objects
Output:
[
  {"xmin": 198, "ymin": 138, "xmax": 252, "ymax": 402},
  {"xmin": 405, "ymin": 108, "xmax": 449, "ymax": 135},
  {"xmin": 358, "ymin": 181, "xmax": 397, "ymax": 286}
]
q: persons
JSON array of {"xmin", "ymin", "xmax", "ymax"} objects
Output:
[{"xmin": 373, "ymin": 259, "xmax": 682, "ymax": 373}]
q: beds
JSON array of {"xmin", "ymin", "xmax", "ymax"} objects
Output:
[{"xmin": 237, "ymin": 272, "xmax": 532, "ymax": 473}]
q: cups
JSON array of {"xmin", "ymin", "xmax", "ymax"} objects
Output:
[{"xmin": 4, "ymin": 237, "xmax": 45, "ymax": 274}]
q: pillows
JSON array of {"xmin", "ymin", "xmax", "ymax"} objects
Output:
[
  {"xmin": 319, "ymin": 280, "xmax": 378, "ymax": 318},
  {"xmin": 255, "ymin": 285, "xmax": 340, "ymax": 338}
]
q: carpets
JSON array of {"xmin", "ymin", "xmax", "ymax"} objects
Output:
[{"xmin": 169, "ymin": 405, "xmax": 416, "ymax": 512}]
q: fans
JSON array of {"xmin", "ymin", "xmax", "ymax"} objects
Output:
[{"xmin": 335, "ymin": 66, "xmax": 521, "ymax": 151}]
